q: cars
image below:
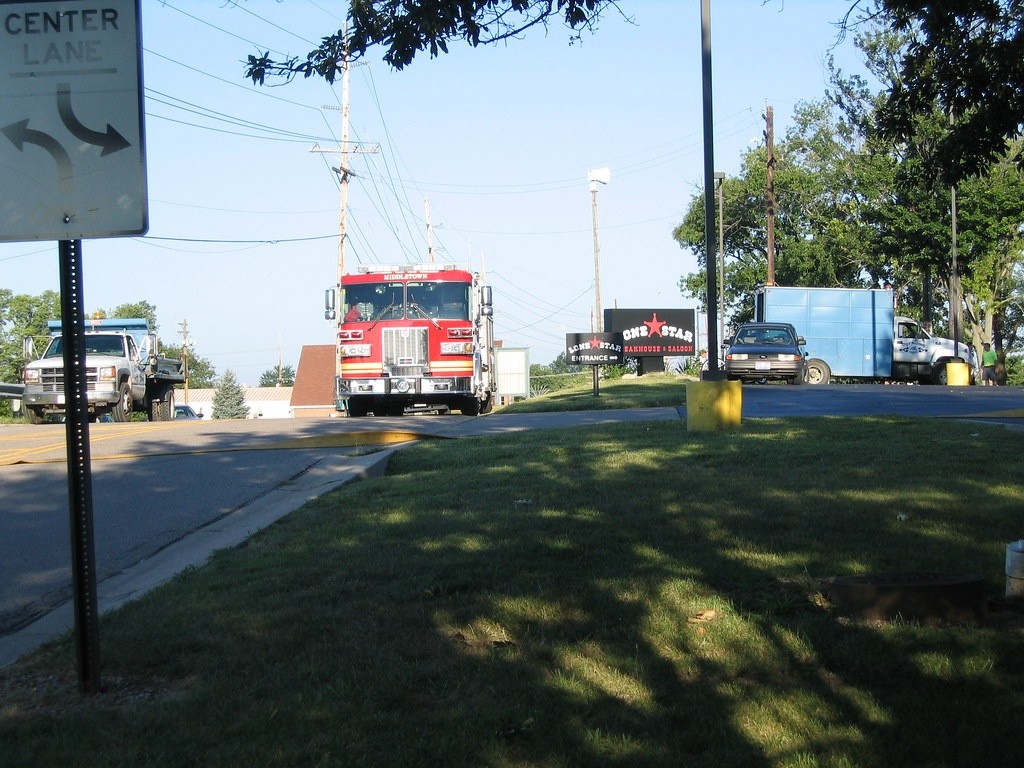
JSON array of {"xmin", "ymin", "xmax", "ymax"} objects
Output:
[
  {"xmin": 174, "ymin": 406, "xmax": 203, "ymax": 420},
  {"xmin": 723, "ymin": 324, "xmax": 806, "ymax": 386}
]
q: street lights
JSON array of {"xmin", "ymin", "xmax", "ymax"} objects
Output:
[{"xmin": 714, "ymin": 173, "xmax": 723, "ymax": 371}]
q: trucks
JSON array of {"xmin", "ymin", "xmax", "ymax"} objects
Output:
[
  {"xmin": 754, "ymin": 287, "xmax": 977, "ymax": 387},
  {"xmin": 21, "ymin": 321, "xmax": 184, "ymax": 424}
]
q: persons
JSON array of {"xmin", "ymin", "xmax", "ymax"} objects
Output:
[{"xmin": 980, "ymin": 342, "xmax": 999, "ymax": 387}]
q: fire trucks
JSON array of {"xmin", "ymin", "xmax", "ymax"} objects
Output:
[{"xmin": 326, "ymin": 260, "xmax": 499, "ymax": 417}]
